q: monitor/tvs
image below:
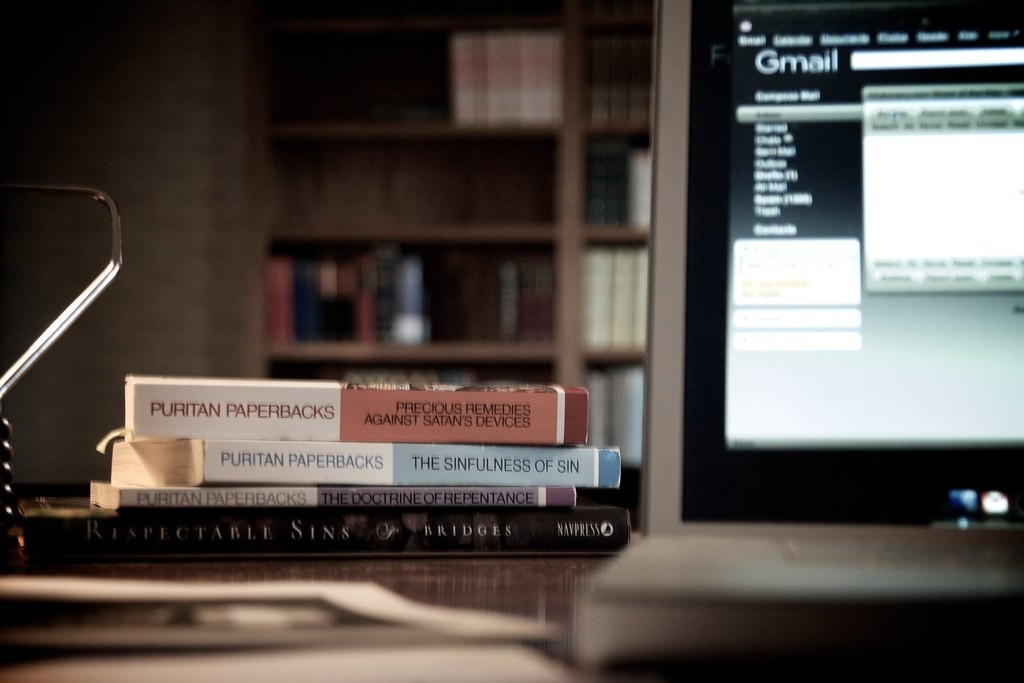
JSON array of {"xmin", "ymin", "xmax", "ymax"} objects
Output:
[{"xmin": 643, "ymin": 0, "xmax": 1024, "ymax": 539}]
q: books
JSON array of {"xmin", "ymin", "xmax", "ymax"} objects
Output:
[{"xmin": 24, "ymin": 374, "xmax": 632, "ymax": 562}]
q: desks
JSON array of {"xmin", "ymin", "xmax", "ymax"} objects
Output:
[{"xmin": 0, "ymin": 552, "xmax": 1024, "ymax": 683}]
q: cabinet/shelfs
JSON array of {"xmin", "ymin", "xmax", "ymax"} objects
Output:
[{"xmin": 245, "ymin": 2, "xmax": 657, "ymax": 513}]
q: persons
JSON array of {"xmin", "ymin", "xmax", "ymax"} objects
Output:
[{"xmin": 268, "ymin": 29, "xmax": 650, "ymax": 465}]
